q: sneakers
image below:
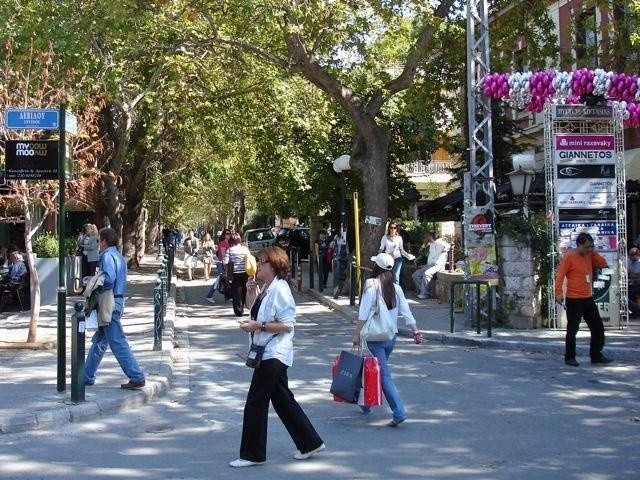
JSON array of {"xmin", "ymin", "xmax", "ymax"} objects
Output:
[
  {"xmin": 204, "ymin": 298, "xmax": 215, "ymax": 304},
  {"xmin": 417, "ymin": 294, "xmax": 429, "ymax": 299},
  {"xmin": 294, "ymin": 443, "xmax": 325, "ymax": 459},
  {"xmin": 229, "ymin": 458, "xmax": 264, "ymax": 467}
]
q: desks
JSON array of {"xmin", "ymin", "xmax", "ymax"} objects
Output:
[
  {"xmin": 450, "ymin": 275, "xmax": 499, "ymax": 337},
  {"xmin": 628, "ymin": 278, "xmax": 640, "ymax": 319}
]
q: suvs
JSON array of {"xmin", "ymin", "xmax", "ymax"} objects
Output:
[{"xmin": 275, "ymin": 227, "xmax": 310, "ymax": 261}]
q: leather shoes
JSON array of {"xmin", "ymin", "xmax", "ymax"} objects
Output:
[
  {"xmin": 591, "ymin": 356, "xmax": 613, "ymax": 363},
  {"xmin": 121, "ymin": 381, "xmax": 145, "ymax": 389},
  {"xmin": 566, "ymin": 359, "xmax": 579, "ymax": 366}
]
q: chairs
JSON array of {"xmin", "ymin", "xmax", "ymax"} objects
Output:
[{"xmin": 0, "ymin": 250, "xmax": 30, "ymax": 312}]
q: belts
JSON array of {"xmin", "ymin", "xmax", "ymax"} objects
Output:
[{"xmin": 114, "ymin": 295, "xmax": 123, "ymax": 298}]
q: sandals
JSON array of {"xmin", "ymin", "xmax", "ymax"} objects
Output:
[{"xmin": 388, "ymin": 418, "xmax": 404, "ymax": 426}]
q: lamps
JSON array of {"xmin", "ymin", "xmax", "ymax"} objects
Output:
[{"xmin": 505, "ymin": 165, "xmax": 535, "ymax": 221}]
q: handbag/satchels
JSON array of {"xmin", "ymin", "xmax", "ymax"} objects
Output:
[
  {"xmin": 222, "ymin": 254, "xmax": 260, "ymax": 309},
  {"xmin": 246, "ymin": 343, "xmax": 265, "ymax": 368},
  {"xmin": 330, "ymin": 343, "xmax": 382, "ymax": 406}
]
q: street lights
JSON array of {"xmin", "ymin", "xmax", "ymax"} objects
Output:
[{"xmin": 333, "ymin": 155, "xmax": 362, "ymax": 297}]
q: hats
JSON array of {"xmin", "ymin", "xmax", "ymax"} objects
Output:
[{"xmin": 371, "ymin": 253, "xmax": 395, "ymax": 270}]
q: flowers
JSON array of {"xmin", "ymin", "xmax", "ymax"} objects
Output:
[{"xmin": 468, "ymin": 246, "xmax": 488, "ymax": 275}]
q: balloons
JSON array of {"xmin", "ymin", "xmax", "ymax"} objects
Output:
[{"xmin": 484, "ymin": 68, "xmax": 640, "ymax": 129}]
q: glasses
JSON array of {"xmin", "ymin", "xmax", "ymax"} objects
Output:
[{"xmin": 390, "ymin": 227, "xmax": 397, "ymax": 229}]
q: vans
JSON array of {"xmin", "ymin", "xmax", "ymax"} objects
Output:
[{"xmin": 244, "ymin": 226, "xmax": 276, "ymax": 257}]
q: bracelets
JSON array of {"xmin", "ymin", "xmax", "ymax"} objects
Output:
[{"xmin": 261, "ymin": 322, "xmax": 266, "ymax": 332}]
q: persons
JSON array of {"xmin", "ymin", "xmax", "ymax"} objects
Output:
[
  {"xmin": 554, "ymin": 232, "xmax": 613, "ymax": 367},
  {"xmin": 0, "ymin": 246, "xmax": 31, "ymax": 312},
  {"xmin": 229, "ymin": 245, "xmax": 325, "ymax": 468},
  {"xmin": 162, "ymin": 220, "xmax": 453, "ymax": 317},
  {"xmin": 79, "ymin": 224, "xmax": 101, "ymax": 292},
  {"xmin": 75, "ymin": 223, "xmax": 91, "ymax": 295},
  {"xmin": 351, "ymin": 252, "xmax": 424, "ymax": 426},
  {"xmin": 619, "ymin": 247, "xmax": 640, "ymax": 320},
  {"xmin": 84, "ymin": 228, "xmax": 145, "ymax": 390}
]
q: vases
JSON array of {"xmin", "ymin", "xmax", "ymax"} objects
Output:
[{"xmin": 472, "ymin": 261, "xmax": 482, "ymax": 276}]
